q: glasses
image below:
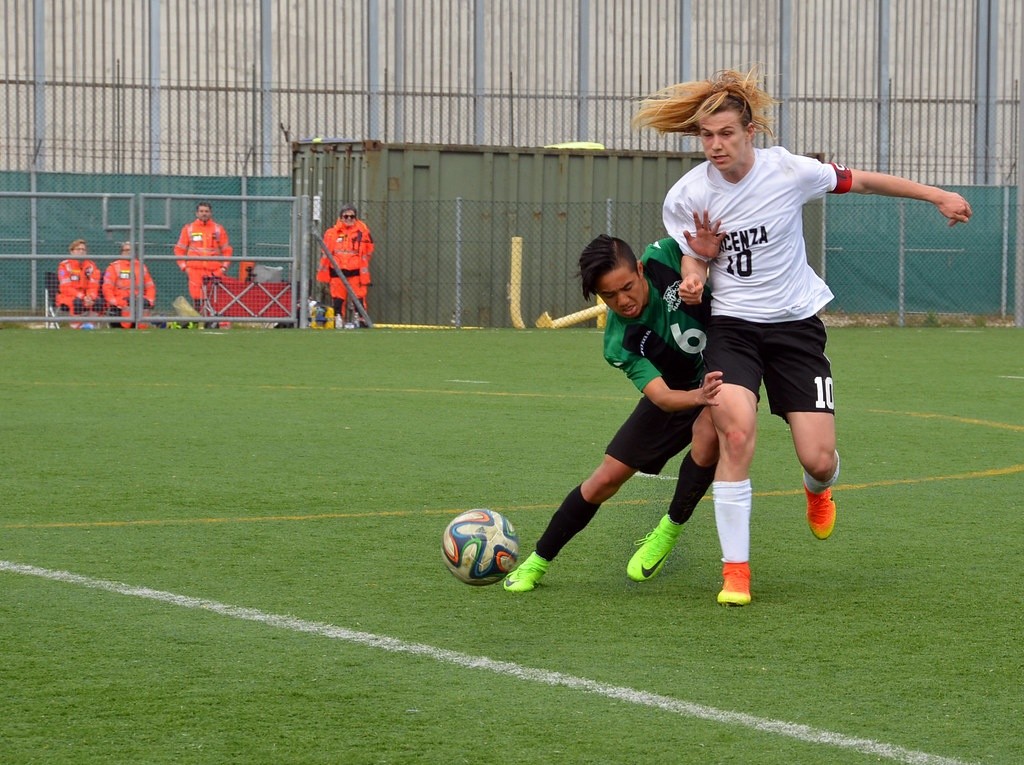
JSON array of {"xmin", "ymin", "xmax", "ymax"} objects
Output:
[{"xmin": 343, "ymin": 214, "xmax": 355, "ymax": 219}]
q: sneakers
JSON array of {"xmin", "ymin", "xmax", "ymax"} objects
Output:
[
  {"xmin": 503, "ymin": 551, "xmax": 551, "ymax": 592},
  {"xmin": 626, "ymin": 514, "xmax": 687, "ymax": 582},
  {"xmin": 802, "ymin": 472, "xmax": 836, "ymax": 540},
  {"xmin": 717, "ymin": 562, "xmax": 751, "ymax": 605}
]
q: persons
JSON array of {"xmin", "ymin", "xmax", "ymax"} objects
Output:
[
  {"xmin": 56, "ymin": 240, "xmax": 105, "ymax": 329},
  {"xmin": 175, "ymin": 202, "xmax": 232, "ymax": 328},
  {"xmin": 317, "ymin": 205, "xmax": 374, "ymax": 328},
  {"xmin": 102, "ymin": 243, "xmax": 156, "ymax": 329},
  {"xmin": 504, "ymin": 209, "xmax": 726, "ymax": 592},
  {"xmin": 633, "ymin": 72, "xmax": 973, "ymax": 606}
]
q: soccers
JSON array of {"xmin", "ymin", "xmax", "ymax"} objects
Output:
[{"xmin": 441, "ymin": 508, "xmax": 520, "ymax": 586}]
